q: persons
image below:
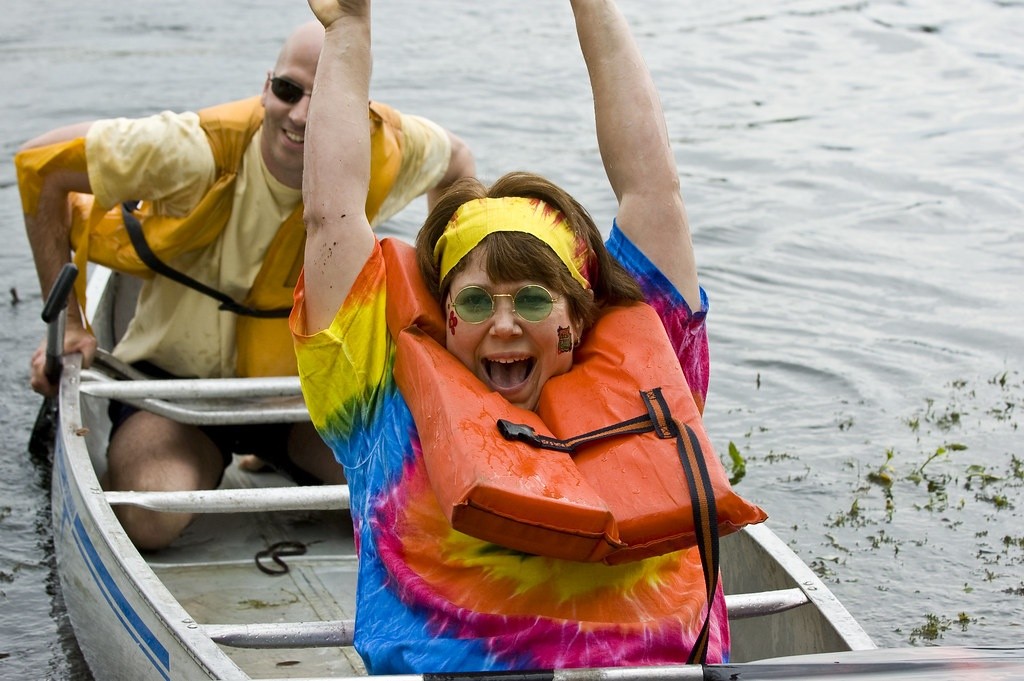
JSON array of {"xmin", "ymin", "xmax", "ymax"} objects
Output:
[
  {"xmin": 14, "ymin": 21, "xmax": 477, "ymax": 550},
  {"xmin": 288, "ymin": 0, "xmax": 731, "ymax": 675}
]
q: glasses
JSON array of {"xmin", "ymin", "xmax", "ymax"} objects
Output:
[
  {"xmin": 449, "ymin": 284, "xmax": 564, "ymax": 324},
  {"xmin": 270, "ymin": 77, "xmax": 311, "ymax": 104}
]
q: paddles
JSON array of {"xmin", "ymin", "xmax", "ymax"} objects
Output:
[
  {"xmin": 322, "ymin": 645, "xmax": 1024, "ymax": 680},
  {"xmin": 28, "ymin": 262, "xmax": 79, "ymax": 457}
]
